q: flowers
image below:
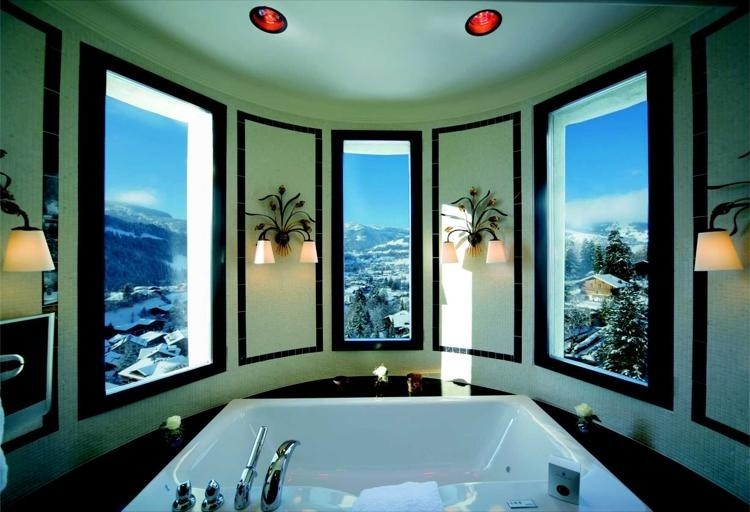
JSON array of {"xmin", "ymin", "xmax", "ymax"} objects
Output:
[
  {"xmin": 575, "ymin": 403, "xmax": 602, "ymax": 426},
  {"xmin": 166, "ymin": 416, "xmax": 183, "ymax": 440}
]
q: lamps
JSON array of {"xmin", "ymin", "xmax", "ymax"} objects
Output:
[
  {"xmin": 245, "ymin": 185, "xmax": 320, "ymax": 265},
  {"xmin": 440, "ymin": 187, "xmax": 507, "ymax": 265},
  {"xmin": 1, "ymin": 149, "xmax": 58, "ymax": 273},
  {"xmin": 693, "ymin": 153, "xmax": 750, "ymax": 272}
]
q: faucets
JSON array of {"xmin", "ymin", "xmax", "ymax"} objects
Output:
[
  {"xmin": 261, "ymin": 438, "xmax": 299, "ymax": 510},
  {"xmin": 230, "ymin": 424, "xmax": 269, "ymax": 510}
]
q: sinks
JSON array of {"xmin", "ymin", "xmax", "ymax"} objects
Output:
[{"xmin": 121, "ymin": 394, "xmax": 650, "ymax": 511}]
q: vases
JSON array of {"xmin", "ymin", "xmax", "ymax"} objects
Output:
[{"xmin": 577, "ymin": 421, "xmax": 595, "ymax": 438}]
